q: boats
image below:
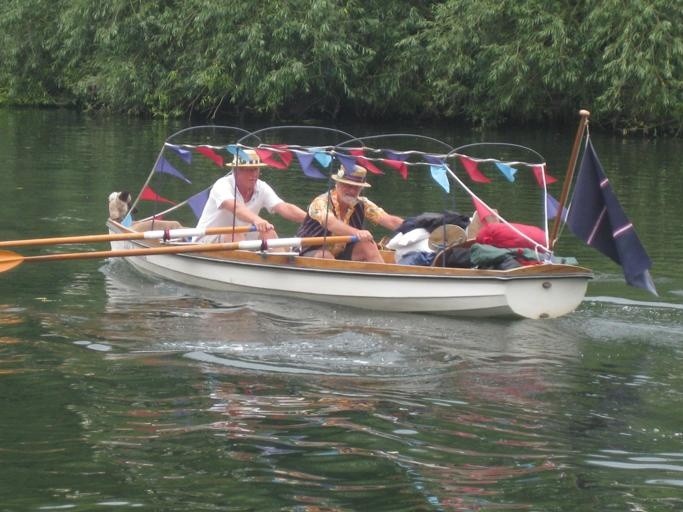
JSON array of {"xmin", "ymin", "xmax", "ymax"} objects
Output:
[{"xmin": 105, "ymin": 126, "xmax": 596, "ymax": 319}]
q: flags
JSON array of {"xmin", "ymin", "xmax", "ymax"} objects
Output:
[{"xmin": 564, "ymin": 135, "xmax": 659, "ymax": 298}]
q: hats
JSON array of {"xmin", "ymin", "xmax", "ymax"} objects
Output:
[
  {"xmin": 330, "ymin": 163, "xmax": 372, "ymax": 188},
  {"xmin": 222, "ymin": 149, "xmax": 268, "ymax": 168}
]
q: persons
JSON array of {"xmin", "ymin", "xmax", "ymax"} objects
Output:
[
  {"xmin": 191, "ymin": 149, "xmax": 306, "ymax": 252},
  {"xmin": 290, "ymin": 163, "xmax": 405, "ymax": 265}
]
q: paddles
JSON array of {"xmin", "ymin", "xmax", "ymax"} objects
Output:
[{"xmin": 0, "ymin": 235, "xmax": 360, "ymax": 273}]
